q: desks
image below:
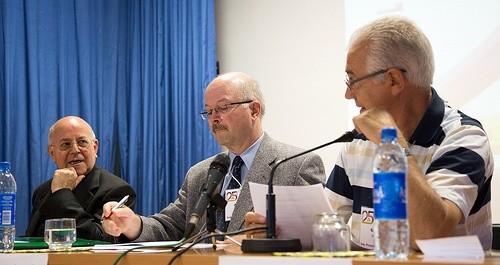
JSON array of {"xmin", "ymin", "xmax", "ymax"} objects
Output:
[{"xmin": 0, "ymin": 242, "xmax": 500, "ymax": 265}]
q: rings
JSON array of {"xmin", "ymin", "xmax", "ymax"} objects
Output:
[{"xmin": 251, "ymin": 234, "xmax": 254, "ymax": 238}]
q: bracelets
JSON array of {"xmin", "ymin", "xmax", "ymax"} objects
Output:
[{"xmin": 390, "ymin": 148, "xmax": 413, "ymax": 162}]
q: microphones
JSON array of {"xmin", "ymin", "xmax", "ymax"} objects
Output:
[
  {"xmin": 239, "ymin": 132, "xmax": 355, "ymax": 254},
  {"xmin": 183, "ymin": 153, "xmax": 231, "ymax": 238}
]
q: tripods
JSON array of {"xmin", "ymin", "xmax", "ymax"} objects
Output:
[{"xmin": 171, "ymin": 198, "xmax": 242, "ymax": 252}]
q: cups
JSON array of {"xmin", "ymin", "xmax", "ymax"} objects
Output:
[
  {"xmin": 312, "ymin": 211, "xmax": 351, "ymax": 252},
  {"xmin": 44, "ymin": 218, "xmax": 77, "ymax": 252}
]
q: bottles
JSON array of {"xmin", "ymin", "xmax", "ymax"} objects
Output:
[
  {"xmin": 373, "ymin": 126, "xmax": 409, "ymax": 262},
  {"xmin": 0, "ymin": 161, "xmax": 17, "ymax": 253}
]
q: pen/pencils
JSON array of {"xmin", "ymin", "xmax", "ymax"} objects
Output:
[{"xmin": 101, "ymin": 195, "xmax": 130, "ymax": 224}]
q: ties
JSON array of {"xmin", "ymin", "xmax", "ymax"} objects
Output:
[{"xmin": 202, "ymin": 156, "xmax": 244, "ymax": 241}]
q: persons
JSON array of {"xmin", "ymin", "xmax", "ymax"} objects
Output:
[
  {"xmin": 244, "ymin": 15, "xmax": 494, "ymax": 251},
  {"xmin": 25, "ymin": 116, "xmax": 137, "ymax": 242},
  {"xmin": 102, "ymin": 72, "xmax": 326, "ymax": 244}
]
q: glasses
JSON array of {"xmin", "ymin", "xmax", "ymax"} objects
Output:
[
  {"xmin": 344, "ymin": 66, "xmax": 407, "ymax": 91},
  {"xmin": 199, "ymin": 100, "xmax": 254, "ymax": 121},
  {"xmin": 50, "ymin": 137, "xmax": 94, "ymax": 153}
]
